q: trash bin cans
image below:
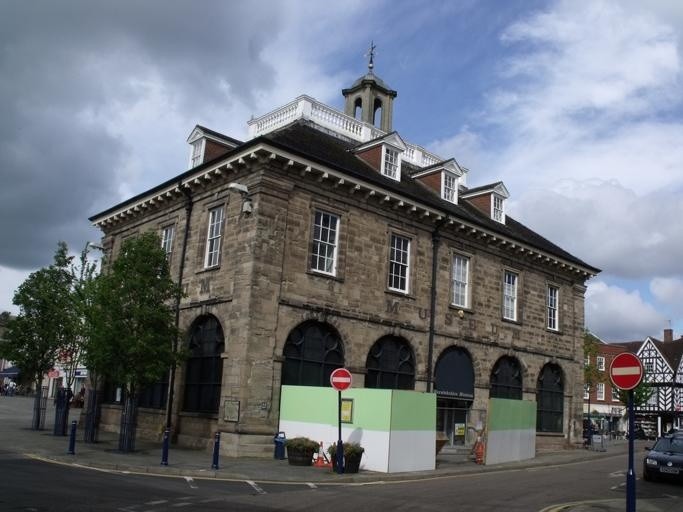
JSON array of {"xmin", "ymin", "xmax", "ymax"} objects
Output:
[
  {"xmin": 274, "ymin": 432, "xmax": 286, "ymax": 460},
  {"xmin": 592, "ymin": 430, "xmax": 608, "ymax": 452}
]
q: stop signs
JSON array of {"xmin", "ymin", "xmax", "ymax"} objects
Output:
[
  {"xmin": 330, "ymin": 368, "xmax": 352, "ymax": 392},
  {"xmin": 608, "ymin": 352, "xmax": 642, "ymax": 392}
]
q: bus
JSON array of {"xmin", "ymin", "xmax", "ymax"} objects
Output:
[{"xmin": 582, "ymin": 418, "xmax": 604, "ymax": 447}]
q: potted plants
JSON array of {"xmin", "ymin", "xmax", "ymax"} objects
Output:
[
  {"xmin": 326, "ymin": 438, "xmax": 364, "ymax": 475},
  {"xmin": 282, "ymin": 437, "xmax": 319, "ymax": 467}
]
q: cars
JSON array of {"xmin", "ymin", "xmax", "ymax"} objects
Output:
[{"xmin": 641, "ymin": 436, "xmax": 682, "ymax": 484}]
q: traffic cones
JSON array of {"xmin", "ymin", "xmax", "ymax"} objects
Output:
[
  {"xmin": 325, "ymin": 442, "xmax": 338, "ymax": 468},
  {"xmin": 313, "ymin": 442, "xmax": 326, "ymax": 469}
]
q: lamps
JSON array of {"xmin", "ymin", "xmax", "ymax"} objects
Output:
[{"xmin": 227, "ymin": 180, "xmax": 253, "ymax": 220}]
